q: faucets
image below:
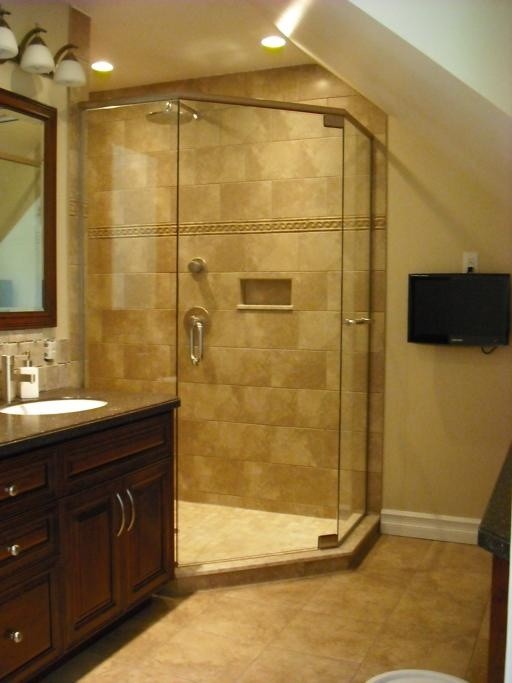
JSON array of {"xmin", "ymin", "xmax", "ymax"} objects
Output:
[{"xmin": 1, "ymin": 354, "xmax": 35, "ymax": 402}]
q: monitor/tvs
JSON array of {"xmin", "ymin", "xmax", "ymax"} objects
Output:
[{"xmin": 406, "ymin": 272, "xmax": 511, "ymax": 346}]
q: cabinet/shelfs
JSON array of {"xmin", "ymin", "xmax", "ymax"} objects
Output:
[
  {"xmin": 0, "ymin": 441, "xmax": 64, "ymax": 683},
  {"xmin": 58, "ymin": 398, "xmax": 180, "ymax": 663},
  {"xmin": 477, "ymin": 441, "xmax": 511, "ymax": 682}
]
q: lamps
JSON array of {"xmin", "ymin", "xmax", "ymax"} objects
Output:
[{"xmin": 0, "ymin": 7, "xmax": 86, "ymax": 87}]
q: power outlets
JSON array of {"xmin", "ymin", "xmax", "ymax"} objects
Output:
[{"xmin": 462, "ymin": 252, "xmax": 480, "ymax": 275}]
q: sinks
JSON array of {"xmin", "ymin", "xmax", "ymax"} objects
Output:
[{"xmin": 1, "ymin": 395, "xmax": 110, "ymax": 414}]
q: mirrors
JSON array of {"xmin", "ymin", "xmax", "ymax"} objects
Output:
[{"xmin": 0, "ymin": 86, "xmax": 58, "ymax": 330}]
